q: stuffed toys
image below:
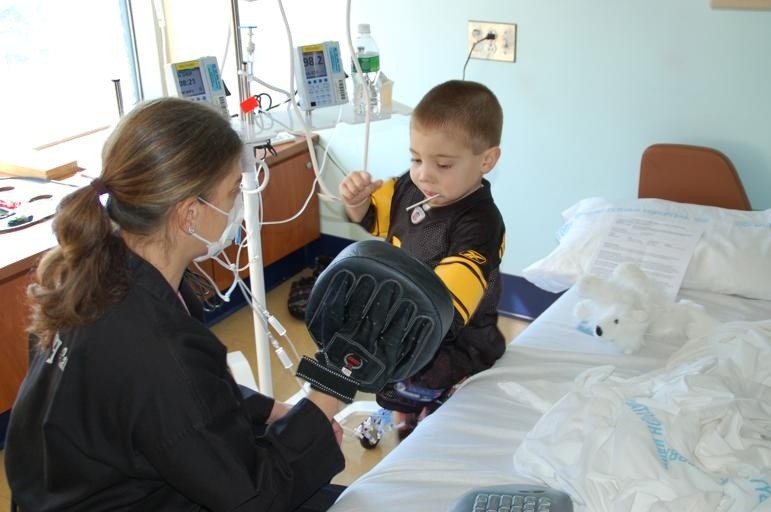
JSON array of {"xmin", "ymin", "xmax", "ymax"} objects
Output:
[{"xmin": 575, "ymin": 262, "xmax": 712, "ymax": 356}]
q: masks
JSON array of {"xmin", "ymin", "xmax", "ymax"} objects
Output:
[{"xmin": 187, "ymin": 193, "xmax": 244, "ymax": 263}]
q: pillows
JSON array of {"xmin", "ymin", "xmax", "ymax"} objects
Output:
[{"xmin": 523, "ymin": 196, "xmax": 771, "ymax": 305}]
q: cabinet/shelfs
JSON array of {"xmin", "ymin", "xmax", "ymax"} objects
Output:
[{"xmin": 1, "ymin": 120, "xmax": 319, "ymax": 418}]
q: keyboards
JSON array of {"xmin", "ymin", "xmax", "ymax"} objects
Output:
[{"xmin": 452, "ymin": 481, "xmax": 573, "ymax": 512}]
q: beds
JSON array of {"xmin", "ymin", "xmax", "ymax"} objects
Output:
[{"xmin": 324, "ymin": 145, "xmax": 771, "ymax": 512}]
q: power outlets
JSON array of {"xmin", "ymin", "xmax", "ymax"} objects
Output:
[{"xmin": 468, "ymin": 22, "xmax": 514, "ymax": 64}]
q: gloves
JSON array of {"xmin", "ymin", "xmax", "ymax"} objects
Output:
[{"xmin": 297, "ymin": 240, "xmax": 455, "ymax": 402}]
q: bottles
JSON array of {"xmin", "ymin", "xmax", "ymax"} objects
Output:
[{"xmin": 351, "ymin": 24, "xmax": 380, "ymax": 117}]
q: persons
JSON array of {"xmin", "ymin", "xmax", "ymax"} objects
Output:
[
  {"xmin": 2, "ymin": 96, "xmax": 349, "ymax": 512},
  {"xmin": 338, "ymin": 79, "xmax": 507, "ymax": 451}
]
